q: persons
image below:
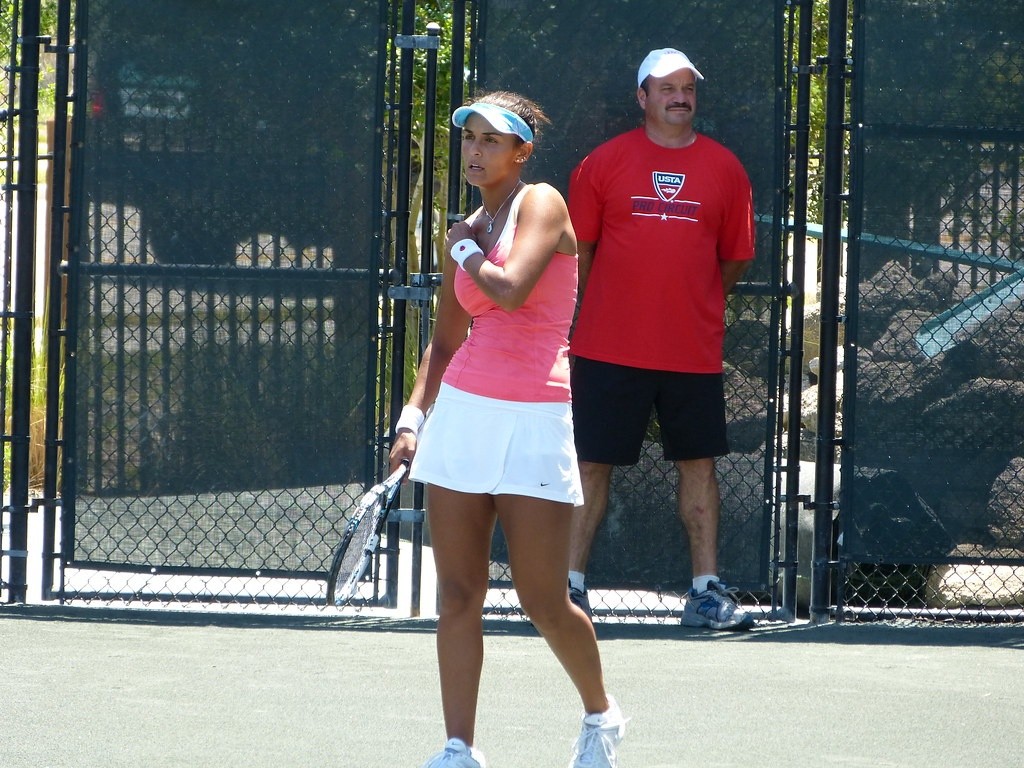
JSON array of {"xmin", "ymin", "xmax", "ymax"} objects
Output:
[
  {"xmin": 389, "ymin": 90, "xmax": 633, "ymax": 768},
  {"xmin": 561, "ymin": 48, "xmax": 758, "ymax": 634}
]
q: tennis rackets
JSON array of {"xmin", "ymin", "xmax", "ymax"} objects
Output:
[{"xmin": 327, "ymin": 404, "xmax": 433, "ymax": 607}]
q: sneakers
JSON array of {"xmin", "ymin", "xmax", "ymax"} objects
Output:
[
  {"xmin": 530, "ymin": 579, "xmax": 593, "ymax": 627},
  {"xmin": 426, "ymin": 738, "xmax": 486, "ymax": 768},
  {"xmin": 568, "ymin": 693, "xmax": 627, "ymax": 768},
  {"xmin": 680, "ymin": 579, "xmax": 753, "ymax": 631}
]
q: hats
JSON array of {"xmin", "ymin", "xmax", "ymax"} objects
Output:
[
  {"xmin": 452, "ymin": 102, "xmax": 534, "ymax": 143},
  {"xmin": 638, "ymin": 48, "xmax": 705, "ymax": 88}
]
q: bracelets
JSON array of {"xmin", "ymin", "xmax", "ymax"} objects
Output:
[
  {"xmin": 450, "ymin": 238, "xmax": 484, "ymax": 272},
  {"xmin": 395, "ymin": 405, "xmax": 426, "ymax": 436}
]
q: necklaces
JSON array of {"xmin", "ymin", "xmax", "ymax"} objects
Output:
[{"xmin": 482, "ymin": 179, "xmax": 522, "ymax": 234}]
